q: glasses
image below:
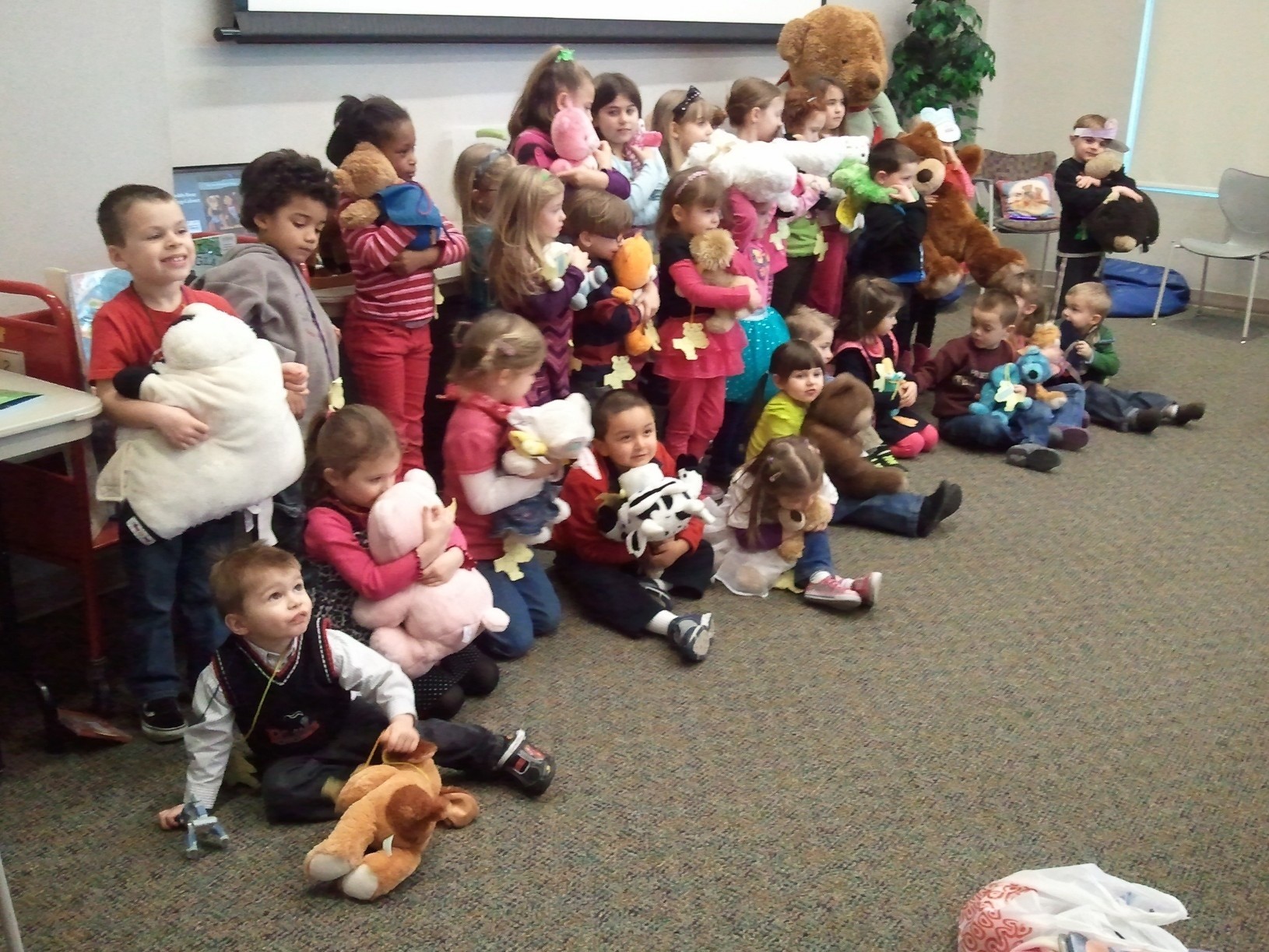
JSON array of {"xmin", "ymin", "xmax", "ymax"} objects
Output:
[{"xmin": 595, "ymin": 233, "xmax": 624, "ymax": 243}]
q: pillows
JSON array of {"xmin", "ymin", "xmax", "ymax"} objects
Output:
[{"xmin": 995, "ymin": 173, "xmax": 1062, "ymax": 222}]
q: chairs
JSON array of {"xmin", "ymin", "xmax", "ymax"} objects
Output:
[
  {"xmin": 970, "ymin": 147, "xmax": 1068, "ymax": 291},
  {"xmin": 1150, "ymin": 165, "xmax": 1269, "ymax": 344}
]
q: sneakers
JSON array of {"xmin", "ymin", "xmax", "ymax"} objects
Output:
[
  {"xmin": 667, "ymin": 611, "xmax": 718, "ymax": 661},
  {"xmin": 140, "ymin": 695, "xmax": 188, "ymax": 742},
  {"xmin": 495, "ymin": 729, "xmax": 555, "ymax": 795},
  {"xmin": 804, "ymin": 575, "xmax": 861, "ymax": 610},
  {"xmin": 852, "ymin": 571, "xmax": 882, "ymax": 607},
  {"xmin": 861, "ymin": 445, "xmax": 909, "ymax": 475}
]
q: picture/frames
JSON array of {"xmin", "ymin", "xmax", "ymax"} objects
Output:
[{"xmin": 172, "ymin": 162, "xmax": 260, "ymax": 238}]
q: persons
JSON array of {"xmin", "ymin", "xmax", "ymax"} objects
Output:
[{"xmin": 81, "ymin": 51, "xmax": 1204, "ymax": 832}]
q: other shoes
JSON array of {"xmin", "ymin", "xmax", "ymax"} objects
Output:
[
  {"xmin": 1128, "ymin": 406, "xmax": 1162, "ymax": 434},
  {"xmin": 1007, "ymin": 442, "xmax": 1060, "ymax": 471},
  {"xmin": 1047, "ymin": 422, "xmax": 1088, "ymax": 451},
  {"xmin": 917, "ymin": 480, "xmax": 963, "ymax": 538},
  {"xmin": 1170, "ymin": 400, "xmax": 1206, "ymax": 426},
  {"xmin": 1080, "ymin": 411, "xmax": 1089, "ymax": 427}
]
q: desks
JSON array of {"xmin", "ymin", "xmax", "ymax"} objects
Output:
[
  {"xmin": 314, "ymin": 260, "xmax": 468, "ymax": 329},
  {"xmin": 0, "ymin": 362, "xmax": 107, "ymax": 695}
]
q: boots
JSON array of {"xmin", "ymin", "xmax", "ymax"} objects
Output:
[
  {"xmin": 897, "ymin": 351, "xmax": 913, "ymax": 380},
  {"xmin": 912, "ymin": 343, "xmax": 933, "ymax": 372}
]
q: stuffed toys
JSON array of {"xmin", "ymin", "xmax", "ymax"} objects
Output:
[
  {"xmin": 893, "ymin": 122, "xmax": 1028, "ymax": 300},
  {"xmin": 777, "ymin": 498, "xmax": 833, "ymax": 562},
  {"xmin": 551, "ymin": 6, "xmax": 918, "ymax": 233},
  {"xmin": 611, "ymin": 231, "xmax": 662, "ymax": 356},
  {"xmin": 674, "ymin": 228, "xmax": 760, "ymax": 334},
  {"xmin": 352, "ymin": 468, "xmax": 511, "ymax": 681},
  {"xmin": 1018, "ymin": 322, "xmax": 1068, "ymax": 410},
  {"xmin": 544, "ymin": 241, "xmax": 608, "ymax": 311},
  {"xmin": 969, "ymin": 346, "xmax": 1052, "ymax": 427},
  {"xmin": 501, "ymin": 392, "xmax": 595, "ymax": 545},
  {"xmin": 1083, "ymin": 148, "xmax": 1159, "ymax": 253},
  {"xmin": 303, "ymin": 740, "xmax": 478, "ymax": 903},
  {"xmin": 334, "ymin": 140, "xmax": 444, "ymax": 252},
  {"xmin": 95, "ymin": 303, "xmax": 305, "ymax": 546},
  {"xmin": 799, "ymin": 373, "xmax": 907, "ymax": 499},
  {"xmin": 594, "ymin": 453, "xmax": 716, "ymax": 559},
  {"xmin": 881, "ymin": 371, "xmax": 905, "ymax": 418}
]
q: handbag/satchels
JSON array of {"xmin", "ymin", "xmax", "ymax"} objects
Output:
[{"xmin": 954, "ymin": 862, "xmax": 1191, "ymax": 950}]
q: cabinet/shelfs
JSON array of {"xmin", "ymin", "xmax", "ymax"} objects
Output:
[
  {"xmin": 0, "ymin": 275, "xmax": 123, "ymax": 714},
  {"xmin": 186, "ymin": 230, "xmax": 313, "ymax": 289}
]
